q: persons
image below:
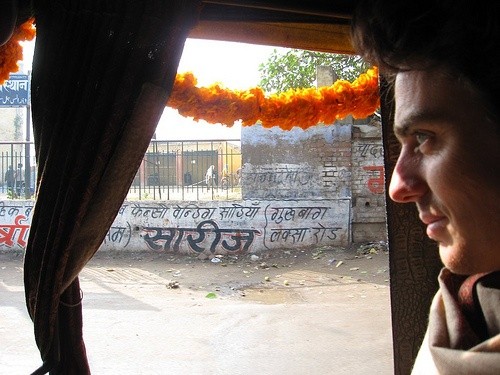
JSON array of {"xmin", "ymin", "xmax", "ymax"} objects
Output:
[
  {"xmin": 349, "ymin": 0, "xmax": 500, "ymax": 375},
  {"xmin": 5, "ymin": 165, "xmax": 15, "ymax": 193},
  {"xmin": 206, "ymin": 166, "xmax": 217, "ymax": 192},
  {"xmin": 14, "ymin": 163, "xmax": 24, "ymax": 196}
]
